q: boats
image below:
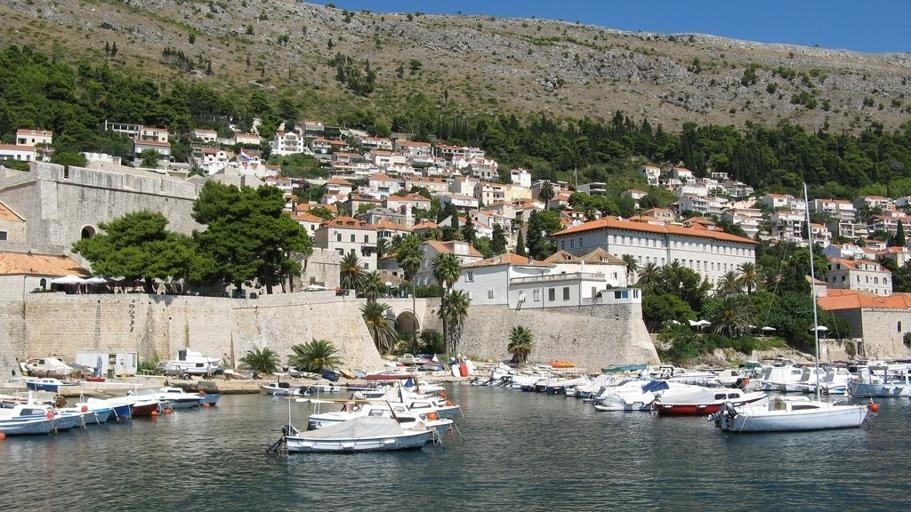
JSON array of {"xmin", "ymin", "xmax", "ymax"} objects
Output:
[
  {"xmin": 0, "ymin": 349, "xmax": 254, "ymax": 435},
  {"xmin": 258, "ymin": 353, "xmax": 475, "ymax": 454}
]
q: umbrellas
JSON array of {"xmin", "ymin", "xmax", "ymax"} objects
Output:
[
  {"xmin": 86, "ymin": 273, "xmax": 118, "ymax": 285},
  {"xmin": 51, "ymin": 275, "xmax": 93, "ymax": 285},
  {"xmin": 760, "ymin": 325, "xmax": 777, "ymax": 331},
  {"xmin": 741, "ymin": 323, "xmax": 757, "ymax": 330},
  {"xmin": 698, "ymin": 319, "xmax": 713, "ymax": 325},
  {"xmin": 809, "ymin": 325, "xmax": 829, "ymax": 332},
  {"xmin": 662, "ymin": 319, "xmax": 682, "ymax": 326},
  {"xmin": 682, "ymin": 319, "xmax": 700, "ymax": 326}
]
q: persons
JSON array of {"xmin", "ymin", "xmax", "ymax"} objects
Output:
[
  {"xmin": 450, "ymin": 351, "xmax": 464, "ymax": 366},
  {"xmin": 76, "ymin": 282, "xmax": 83, "ymax": 295}
]
